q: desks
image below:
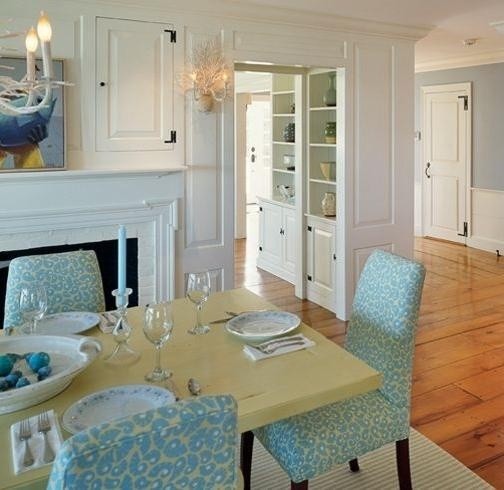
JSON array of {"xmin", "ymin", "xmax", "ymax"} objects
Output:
[{"xmin": 3, "ymin": 265, "xmax": 380, "ymax": 486}]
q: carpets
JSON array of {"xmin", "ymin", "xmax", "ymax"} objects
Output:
[{"xmin": 238, "ymin": 425, "xmax": 498, "ymax": 490}]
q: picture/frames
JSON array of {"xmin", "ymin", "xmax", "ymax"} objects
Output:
[{"xmin": 0, "ymin": 55, "xmax": 68, "ymax": 172}]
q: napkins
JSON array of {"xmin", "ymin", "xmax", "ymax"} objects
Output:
[
  {"xmin": 9, "ymin": 409, "xmax": 67, "ymax": 474},
  {"xmin": 97, "ymin": 311, "xmax": 122, "ymax": 334},
  {"xmin": 243, "ymin": 333, "xmax": 316, "ymax": 361}
]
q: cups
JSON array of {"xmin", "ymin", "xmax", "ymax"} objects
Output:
[{"xmin": 20, "ymin": 286, "xmax": 49, "ymax": 336}]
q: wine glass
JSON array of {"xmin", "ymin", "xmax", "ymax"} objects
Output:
[
  {"xmin": 184, "ymin": 268, "xmax": 212, "ymax": 336},
  {"xmin": 143, "ymin": 301, "xmax": 174, "ymax": 384}
]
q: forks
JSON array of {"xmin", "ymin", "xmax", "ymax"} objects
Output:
[
  {"xmin": 245, "ymin": 337, "xmax": 304, "ymax": 349},
  {"xmin": 37, "ymin": 408, "xmax": 55, "ymax": 463},
  {"xmin": 256, "ymin": 341, "xmax": 305, "ymax": 355},
  {"xmin": 18, "ymin": 416, "xmax": 36, "ymax": 468}
]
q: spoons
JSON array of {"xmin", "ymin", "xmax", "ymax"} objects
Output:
[{"xmin": 187, "ymin": 377, "xmax": 201, "ymax": 397}]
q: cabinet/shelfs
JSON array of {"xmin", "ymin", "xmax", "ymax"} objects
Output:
[
  {"xmin": 257, "ymin": 68, "xmax": 337, "ymax": 314},
  {"xmin": 67, "ymin": 11, "xmax": 187, "ymax": 168}
]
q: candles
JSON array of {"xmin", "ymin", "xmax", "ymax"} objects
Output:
[{"xmin": 118, "ymin": 223, "xmax": 127, "ymax": 306}]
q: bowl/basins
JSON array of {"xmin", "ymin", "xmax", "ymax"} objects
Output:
[{"xmin": 318, "ymin": 160, "xmax": 336, "ymax": 182}]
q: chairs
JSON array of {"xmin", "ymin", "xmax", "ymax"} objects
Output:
[
  {"xmin": 239, "ymin": 249, "xmax": 426, "ymax": 490},
  {"xmin": 1, "ymin": 250, "xmax": 105, "ymax": 329},
  {"xmin": 48, "ymin": 392, "xmax": 240, "ymax": 490}
]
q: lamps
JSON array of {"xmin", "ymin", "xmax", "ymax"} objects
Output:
[
  {"xmin": 176, "ymin": 38, "xmax": 232, "ymax": 113},
  {"xmin": 1, "ymin": 8, "xmax": 77, "ymax": 117}
]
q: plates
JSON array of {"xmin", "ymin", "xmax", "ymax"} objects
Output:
[
  {"xmin": 0, "ymin": 334, "xmax": 105, "ymax": 418},
  {"xmin": 15, "ymin": 310, "xmax": 101, "ymax": 338},
  {"xmin": 225, "ymin": 309, "xmax": 302, "ymax": 345},
  {"xmin": 59, "ymin": 383, "xmax": 177, "ymax": 437}
]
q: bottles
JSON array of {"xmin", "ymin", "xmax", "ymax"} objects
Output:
[
  {"xmin": 321, "ymin": 192, "xmax": 337, "ymax": 218},
  {"xmin": 324, "ymin": 122, "xmax": 337, "ymax": 143},
  {"xmin": 321, "ymin": 73, "xmax": 336, "ymax": 108}
]
